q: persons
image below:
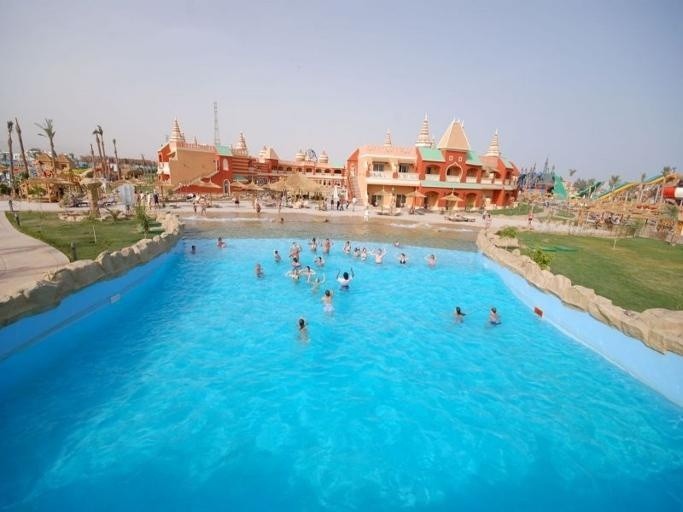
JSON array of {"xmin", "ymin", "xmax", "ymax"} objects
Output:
[
  {"xmin": 528, "ymin": 212, "xmax": 533, "ymax": 229},
  {"xmin": 297, "ymin": 318, "xmax": 309, "ymax": 343},
  {"xmin": 453, "ymin": 306, "xmax": 502, "ymax": 328},
  {"xmin": 482, "ymin": 210, "xmax": 491, "ymax": 226},
  {"xmin": 215, "ymin": 236, "xmax": 225, "ymax": 248},
  {"xmin": 134, "ymin": 189, "xmax": 357, "ymax": 217},
  {"xmin": 189, "ymin": 245, "xmax": 198, "ymax": 255},
  {"xmin": 363, "ymin": 205, "xmax": 369, "ymax": 223},
  {"xmin": 254, "ymin": 237, "xmax": 438, "ymax": 314}
]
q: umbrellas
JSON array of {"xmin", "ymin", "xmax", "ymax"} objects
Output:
[
  {"xmin": 440, "ymin": 192, "xmax": 464, "ymax": 210},
  {"xmin": 173, "ymin": 173, "xmax": 327, "ymax": 204},
  {"xmin": 372, "ymin": 190, "xmax": 393, "ymax": 205},
  {"xmin": 405, "ymin": 190, "xmax": 428, "ymax": 206}
]
